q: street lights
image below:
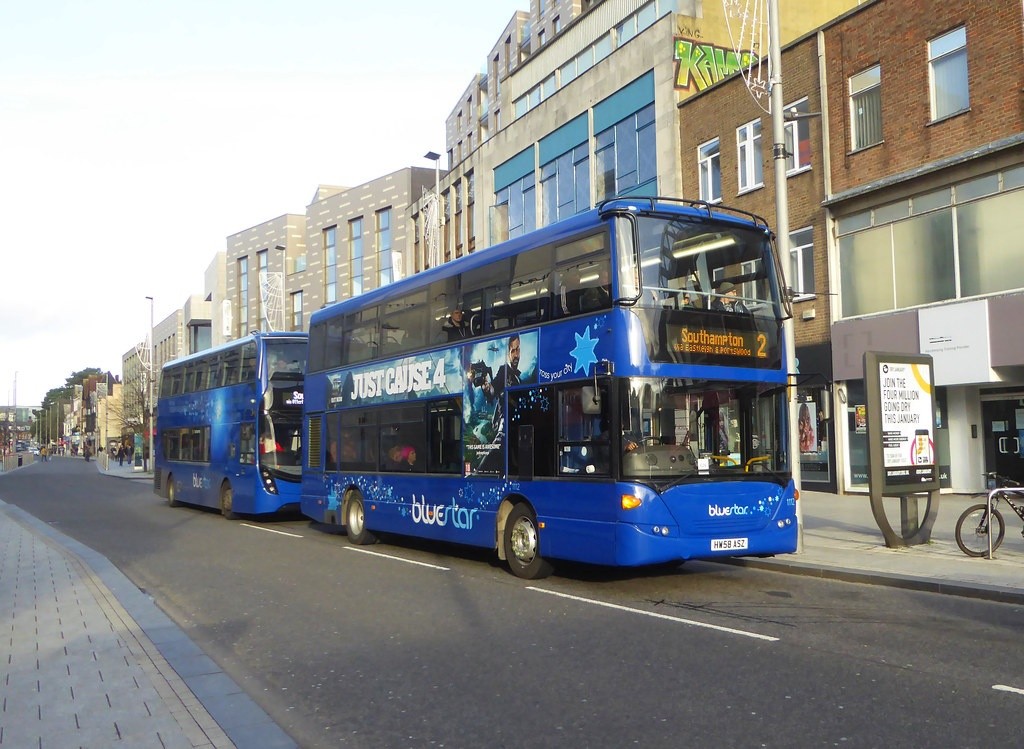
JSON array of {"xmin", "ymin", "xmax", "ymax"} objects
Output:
[
  {"xmin": 275, "ymin": 245, "xmax": 286, "ymax": 331},
  {"xmin": 145, "ymin": 296, "xmax": 154, "ymax": 474},
  {"xmin": 423, "ymin": 152, "xmax": 441, "ymax": 268}
]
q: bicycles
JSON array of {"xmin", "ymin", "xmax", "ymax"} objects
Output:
[{"xmin": 955, "ymin": 472, "xmax": 1024, "ymax": 557}]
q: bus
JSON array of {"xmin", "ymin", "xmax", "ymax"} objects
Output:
[
  {"xmin": 153, "ymin": 330, "xmax": 366, "ymax": 520},
  {"xmin": 300, "ymin": 196, "xmax": 832, "ymax": 581}
]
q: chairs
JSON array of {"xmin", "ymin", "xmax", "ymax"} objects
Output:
[
  {"xmin": 382, "ymin": 336, "xmax": 401, "ymax": 357},
  {"xmin": 471, "ymin": 315, "xmax": 482, "ymax": 335},
  {"xmin": 363, "ymin": 341, "xmax": 378, "ymax": 359}
]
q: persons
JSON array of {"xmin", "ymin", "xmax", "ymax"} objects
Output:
[
  {"xmin": 595, "ymin": 410, "xmax": 640, "ymax": 463},
  {"xmin": 82, "ymin": 443, "xmax": 134, "ymax": 467},
  {"xmin": 251, "ymin": 428, "xmax": 286, "ymax": 464},
  {"xmin": 330, "ymin": 425, "xmax": 420, "ymax": 472},
  {"xmin": 474, "ymin": 331, "xmax": 523, "ymax": 474},
  {"xmin": 441, "ymin": 303, "xmax": 475, "ymax": 343},
  {"xmin": 711, "ymin": 281, "xmax": 749, "ymax": 312},
  {"xmin": 40, "ymin": 444, "xmax": 78, "ymax": 462},
  {"xmin": 565, "ymin": 267, "xmax": 610, "ymax": 315}
]
